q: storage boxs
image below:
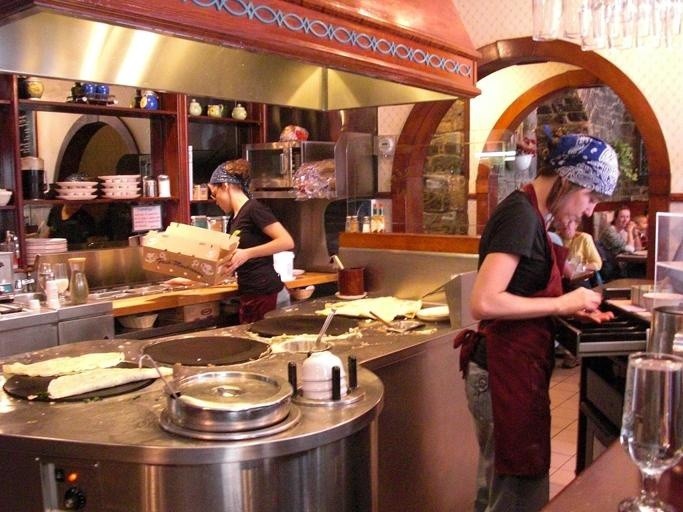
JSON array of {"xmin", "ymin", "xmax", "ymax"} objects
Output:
[{"xmin": 141, "ymin": 221, "xmax": 240, "ymax": 286}]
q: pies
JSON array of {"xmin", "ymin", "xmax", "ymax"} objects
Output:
[
  {"xmin": 3, "ymin": 352, "xmax": 123, "ymax": 377},
  {"xmin": 48, "ymin": 367, "xmax": 173, "ymax": 399}
]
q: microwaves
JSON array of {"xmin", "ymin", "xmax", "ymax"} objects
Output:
[{"xmin": 241, "ymin": 140, "xmax": 336, "ymax": 190}]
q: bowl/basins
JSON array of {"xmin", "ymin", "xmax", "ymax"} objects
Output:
[
  {"xmin": 291, "ymin": 286, "xmax": 315, "ymax": 300},
  {"xmin": 301, "ymin": 349, "xmax": 347, "ymax": 403},
  {"xmin": 1, "ymin": 190, "xmax": 13, "ymax": 206},
  {"xmin": 642, "ymin": 293, "xmax": 683, "ymax": 312}
]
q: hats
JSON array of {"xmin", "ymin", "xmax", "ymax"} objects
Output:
[
  {"xmin": 210, "ymin": 162, "xmax": 249, "ymax": 185},
  {"xmin": 546, "ymin": 133, "xmax": 620, "ymax": 197}
]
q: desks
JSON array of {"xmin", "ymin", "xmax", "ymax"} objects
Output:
[{"xmin": 88, "ymin": 269, "xmax": 339, "ymax": 341}]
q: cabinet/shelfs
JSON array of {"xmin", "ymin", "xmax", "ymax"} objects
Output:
[
  {"xmin": 242, "ymin": 132, "xmax": 374, "ymax": 273},
  {"xmin": 0, "ymin": 70, "xmax": 267, "ymax": 293},
  {"xmin": 575, "ymin": 357, "xmax": 628, "ymax": 476},
  {"xmin": 0, "ymin": 296, "xmax": 115, "ymax": 358}
]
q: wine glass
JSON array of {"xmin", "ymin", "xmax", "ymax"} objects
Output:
[
  {"xmin": 38, "ymin": 262, "xmax": 55, "ymax": 305},
  {"xmin": 53, "ymin": 262, "xmax": 69, "ymax": 304},
  {"xmin": 617, "ymin": 352, "xmax": 683, "ymax": 512},
  {"xmin": 645, "ymin": 306, "xmax": 682, "ymax": 353}
]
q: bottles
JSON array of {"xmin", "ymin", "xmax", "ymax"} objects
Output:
[
  {"xmin": 69, "ymin": 257, "xmax": 89, "ymax": 302},
  {"xmin": 72, "ymin": 81, "xmax": 84, "ymax": 103},
  {"xmin": 372, "ymin": 204, "xmax": 378, "ymax": 233},
  {"xmin": 350, "ymin": 216, "xmax": 358, "ymax": 234},
  {"xmin": 362, "ymin": 217, "xmax": 370, "ymax": 234},
  {"xmin": 379, "ymin": 204, "xmax": 387, "ymax": 233},
  {"xmin": 345, "ymin": 216, "xmax": 351, "ymax": 233}
]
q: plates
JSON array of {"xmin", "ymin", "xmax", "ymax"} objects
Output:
[
  {"xmin": 97, "ymin": 173, "xmax": 141, "ymax": 201},
  {"xmin": 26, "ymin": 238, "xmax": 68, "ymax": 265},
  {"xmin": 335, "ymin": 291, "xmax": 366, "ymax": 300},
  {"xmin": 54, "ymin": 180, "xmax": 98, "ymax": 201},
  {"xmin": 417, "ymin": 305, "xmax": 448, "ymax": 322}
]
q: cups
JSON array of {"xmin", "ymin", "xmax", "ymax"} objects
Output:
[{"xmin": 19, "ymin": 156, "xmax": 50, "ymax": 202}]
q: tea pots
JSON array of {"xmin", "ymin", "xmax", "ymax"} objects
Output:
[
  {"xmin": 208, "ymin": 102, "xmax": 224, "ymax": 118},
  {"xmin": 189, "ymin": 98, "xmax": 202, "ymax": 116},
  {"xmin": 232, "ymin": 103, "xmax": 247, "ymax": 120}
]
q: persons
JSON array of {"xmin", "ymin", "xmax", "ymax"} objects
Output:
[
  {"xmin": 454, "ymin": 132, "xmax": 619, "ymax": 512},
  {"xmin": 633, "ymin": 215, "xmax": 648, "ymax": 244},
  {"xmin": 596, "ymin": 205, "xmax": 641, "ymax": 282},
  {"xmin": 554, "ymin": 217, "xmax": 602, "ymax": 293},
  {"xmin": 208, "ymin": 158, "xmax": 294, "ymax": 324},
  {"xmin": 40, "ymin": 175, "xmax": 98, "ymax": 244}
]
